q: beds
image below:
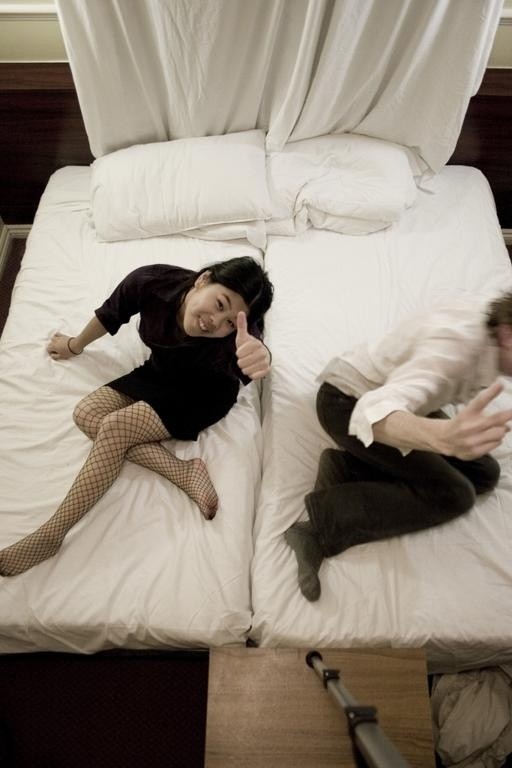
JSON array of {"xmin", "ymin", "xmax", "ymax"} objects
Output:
[
  {"xmin": 0, "ymin": 160, "xmax": 257, "ymax": 653},
  {"xmin": 264, "ymin": 170, "xmax": 512, "ymax": 669}
]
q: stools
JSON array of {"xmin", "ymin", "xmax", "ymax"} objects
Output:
[{"xmin": 204, "ymin": 645, "xmax": 433, "ymax": 768}]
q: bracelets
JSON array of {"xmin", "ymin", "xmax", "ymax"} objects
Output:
[{"xmin": 67, "ymin": 337, "xmax": 85, "ymax": 356}]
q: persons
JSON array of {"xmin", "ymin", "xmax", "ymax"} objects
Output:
[
  {"xmin": 283, "ymin": 288, "xmax": 512, "ymax": 604},
  {"xmin": 0, "ymin": 256, "xmax": 273, "ymax": 578}
]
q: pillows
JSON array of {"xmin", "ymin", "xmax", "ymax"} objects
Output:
[
  {"xmin": 267, "ymin": 135, "xmax": 423, "ymax": 235},
  {"xmin": 90, "ymin": 132, "xmax": 272, "ymax": 245}
]
